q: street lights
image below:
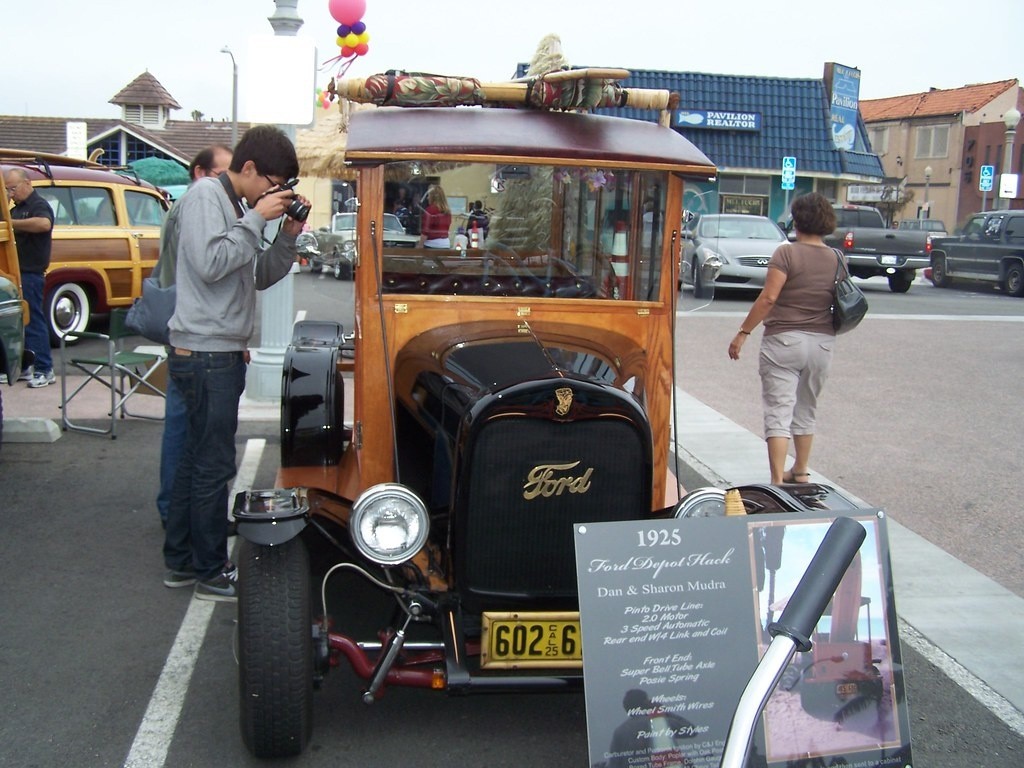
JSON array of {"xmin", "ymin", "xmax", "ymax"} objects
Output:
[
  {"xmin": 924, "ymin": 166, "xmax": 932, "ymax": 219},
  {"xmin": 999, "ymin": 107, "xmax": 1021, "ymax": 210},
  {"xmin": 221, "ymin": 48, "xmax": 238, "ymax": 151}
]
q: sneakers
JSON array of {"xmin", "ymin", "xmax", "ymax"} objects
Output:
[
  {"xmin": 165, "ymin": 567, "xmax": 197, "ymax": 589},
  {"xmin": 196, "ymin": 564, "xmax": 240, "ymax": 602},
  {"xmin": 0, "ymin": 364, "xmax": 33, "ymax": 385},
  {"xmin": 27, "ymin": 368, "xmax": 56, "ymax": 387}
]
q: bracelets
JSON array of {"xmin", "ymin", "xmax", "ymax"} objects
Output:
[{"xmin": 739, "ymin": 328, "xmax": 750, "ymax": 334}]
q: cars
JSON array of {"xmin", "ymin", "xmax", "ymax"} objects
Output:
[
  {"xmin": 237, "ymin": 108, "xmax": 866, "ymax": 757},
  {"xmin": 310, "ymin": 209, "xmax": 421, "ymax": 279},
  {"xmin": 676, "ymin": 214, "xmax": 790, "ymax": 299}
]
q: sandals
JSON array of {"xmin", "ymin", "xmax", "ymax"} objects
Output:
[{"xmin": 782, "ymin": 467, "xmax": 810, "ymax": 484}]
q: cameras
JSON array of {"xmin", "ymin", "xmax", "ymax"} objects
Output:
[{"xmin": 273, "ymin": 178, "xmax": 310, "ymax": 223}]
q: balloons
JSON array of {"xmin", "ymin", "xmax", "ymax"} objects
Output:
[
  {"xmin": 315, "ymin": 87, "xmax": 330, "ymax": 110},
  {"xmin": 328, "ymin": 0, "xmax": 370, "ymax": 57}
]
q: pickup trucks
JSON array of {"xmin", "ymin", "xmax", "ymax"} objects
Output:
[
  {"xmin": 929, "ymin": 209, "xmax": 1024, "ymax": 297},
  {"xmin": 778, "ymin": 204, "xmax": 932, "ymax": 293}
]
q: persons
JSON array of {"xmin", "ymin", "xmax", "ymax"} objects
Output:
[
  {"xmin": 418, "ymin": 186, "xmax": 452, "ymax": 249},
  {"xmin": 610, "ymin": 689, "xmax": 698, "ymax": 768},
  {"xmin": 392, "ymin": 187, "xmax": 421, "ymax": 235},
  {"xmin": 0, "ymin": 168, "xmax": 56, "ymax": 388},
  {"xmin": 465, "ymin": 200, "xmax": 489, "ymax": 242},
  {"xmin": 728, "ymin": 192, "xmax": 849, "ymax": 485},
  {"xmin": 642, "ymin": 200, "xmax": 664, "ymax": 257},
  {"xmin": 156, "ymin": 145, "xmax": 251, "ymax": 537},
  {"xmin": 163, "ymin": 126, "xmax": 312, "ymax": 603},
  {"xmin": 892, "ymin": 221, "xmax": 898, "ymax": 229}
]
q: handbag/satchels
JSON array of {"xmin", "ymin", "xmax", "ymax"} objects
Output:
[
  {"xmin": 831, "ymin": 245, "xmax": 868, "ymax": 335},
  {"xmin": 124, "ymin": 273, "xmax": 179, "ymax": 344}
]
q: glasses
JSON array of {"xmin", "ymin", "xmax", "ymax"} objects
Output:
[
  {"xmin": 5, "ymin": 179, "xmax": 27, "ymax": 194},
  {"xmin": 256, "ymin": 169, "xmax": 282, "ymax": 188}
]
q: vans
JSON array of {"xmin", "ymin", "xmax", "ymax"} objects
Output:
[{"xmin": 896, "ymin": 219, "xmax": 947, "ymax": 238}]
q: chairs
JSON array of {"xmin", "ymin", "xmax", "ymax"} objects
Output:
[{"xmin": 58, "ymin": 308, "xmax": 168, "ymax": 439}]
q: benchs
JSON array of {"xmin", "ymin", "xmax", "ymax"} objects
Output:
[{"xmin": 381, "ymin": 271, "xmax": 597, "ymax": 299}]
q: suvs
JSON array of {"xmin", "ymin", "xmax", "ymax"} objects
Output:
[{"xmin": 0, "ymin": 148, "xmax": 179, "ymax": 439}]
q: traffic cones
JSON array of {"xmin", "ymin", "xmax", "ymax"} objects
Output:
[{"xmin": 599, "ymin": 220, "xmax": 629, "ymax": 300}]
